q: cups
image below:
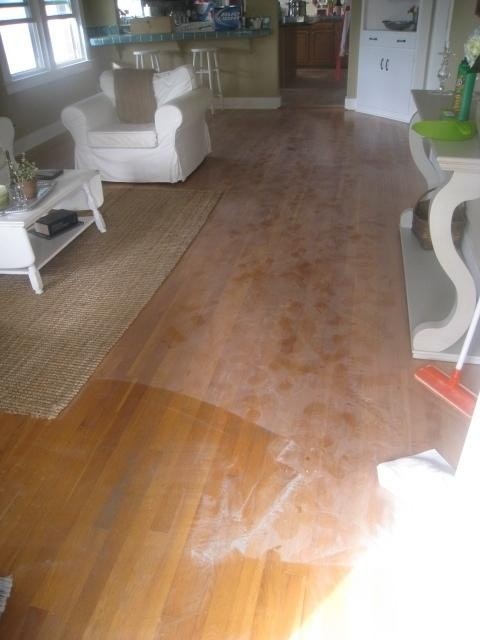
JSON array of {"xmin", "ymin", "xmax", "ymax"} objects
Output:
[{"xmin": 7, "ymin": 183, "xmax": 27, "ymax": 206}]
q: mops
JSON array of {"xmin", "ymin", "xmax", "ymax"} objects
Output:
[{"xmin": 411, "ymin": 294, "xmax": 480, "ymax": 419}]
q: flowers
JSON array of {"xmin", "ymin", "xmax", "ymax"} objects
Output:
[{"xmin": 463, "ymin": 23, "xmax": 480, "ymax": 73}]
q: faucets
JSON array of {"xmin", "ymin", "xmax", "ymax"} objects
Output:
[{"xmin": 407, "ymin": 5, "xmax": 419, "ymax": 22}]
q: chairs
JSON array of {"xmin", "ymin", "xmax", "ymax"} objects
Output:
[{"xmin": 60, "ymin": 62, "xmax": 214, "ymax": 186}]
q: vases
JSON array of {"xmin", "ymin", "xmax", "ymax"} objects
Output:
[{"xmin": 457, "ymin": 60, "xmax": 477, "ymax": 123}]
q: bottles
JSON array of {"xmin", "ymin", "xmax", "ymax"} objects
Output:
[{"xmin": 454, "ymin": 56, "xmax": 470, "ymax": 96}]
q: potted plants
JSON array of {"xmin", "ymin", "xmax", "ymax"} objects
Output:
[{"xmin": 3, "ymin": 145, "xmax": 42, "ymax": 201}]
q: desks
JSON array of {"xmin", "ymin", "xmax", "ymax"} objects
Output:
[
  {"xmin": 395, "ymin": 87, "xmax": 480, "ymax": 365},
  {"xmin": 88, "ymin": 25, "xmax": 275, "ymax": 54}
]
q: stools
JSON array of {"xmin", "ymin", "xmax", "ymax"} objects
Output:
[
  {"xmin": 190, "ymin": 46, "xmax": 225, "ymax": 113},
  {"xmin": 132, "ymin": 47, "xmax": 162, "ymax": 72}
]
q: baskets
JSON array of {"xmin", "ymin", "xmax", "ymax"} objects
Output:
[{"xmin": 412, "ymin": 186, "xmax": 464, "ymax": 252}]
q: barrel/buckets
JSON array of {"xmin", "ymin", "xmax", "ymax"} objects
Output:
[{"xmin": 207, "ymin": 4, "xmax": 241, "ymax": 32}]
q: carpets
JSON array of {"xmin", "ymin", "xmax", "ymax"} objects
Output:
[{"xmin": 0, "ymin": 183, "xmax": 224, "ymax": 422}]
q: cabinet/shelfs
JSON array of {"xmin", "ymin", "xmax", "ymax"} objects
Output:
[
  {"xmin": 295, "ymin": 23, "xmax": 348, "ymax": 70},
  {"xmin": 358, "ymin": 28, "xmax": 419, "ymax": 126}
]
